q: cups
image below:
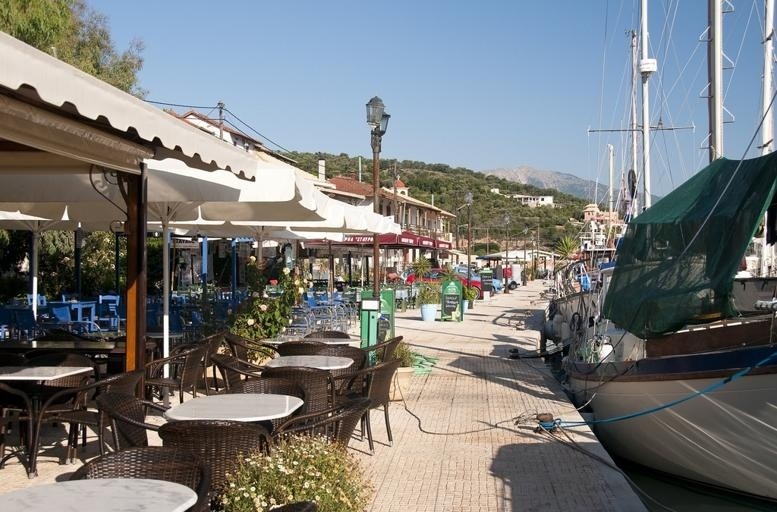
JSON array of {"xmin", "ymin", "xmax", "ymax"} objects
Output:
[{"xmin": 2, "ymin": 326, "xmax": 37, "ymax": 349}]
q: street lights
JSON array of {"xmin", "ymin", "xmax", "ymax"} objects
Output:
[
  {"xmin": 521, "ymin": 223, "xmax": 529, "ymax": 288},
  {"xmin": 501, "ymin": 208, "xmax": 512, "ymax": 294},
  {"xmin": 463, "ymin": 188, "xmax": 476, "ymax": 309},
  {"xmin": 528, "ymin": 234, "xmax": 536, "ymax": 284},
  {"xmin": 363, "ymin": 94, "xmax": 393, "ymax": 321}
]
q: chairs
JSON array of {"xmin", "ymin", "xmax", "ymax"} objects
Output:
[{"xmin": 0, "ymin": 273, "xmax": 420, "ymax": 511}]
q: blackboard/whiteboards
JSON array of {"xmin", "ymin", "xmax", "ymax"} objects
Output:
[
  {"xmin": 480, "ymin": 273, "xmax": 492, "ymax": 291},
  {"xmin": 378, "ymin": 314, "xmax": 390, "ymax": 340},
  {"xmin": 442, "ymin": 293, "xmax": 463, "ymax": 318}
]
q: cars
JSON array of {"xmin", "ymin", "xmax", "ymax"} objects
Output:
[{"xmin": 400, "ymin": 263, "xmax": 520, "ymax": 302}]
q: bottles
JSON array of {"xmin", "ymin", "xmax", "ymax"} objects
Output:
[
  {"xmin": 379, "ymin": 294, "xmax": 392, "ymax": 342},
  {"xmin": 443, "ymin": 281, "xmax": 460, "ymax": 316}
]
